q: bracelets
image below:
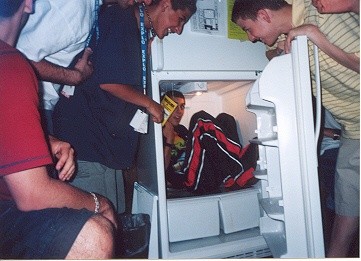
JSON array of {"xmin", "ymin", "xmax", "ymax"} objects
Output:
[{"xmin": 90, "ymin": 191, "xmax": 102, "ymax": 216}]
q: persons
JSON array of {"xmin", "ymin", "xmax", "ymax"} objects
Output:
[
  {"xmin": 0, "ymin": 0, "xmax": 122, "ymax": 260},
  {"xmin": 309, "ymin": 98, "xmax": 346, "ymax": 239},
  {"xmin": 158, "ymin": 90, "xmax": 262, "ymax": 196},
  {"xmin": 62, "ymin": 0, "xmax": 199, "ymax": 233},
  {"xmin": 229, "ymin": 0, "xmax": 359, "ymax": 259},
  {"xmin": 310, "ymin": 0, "xmax": 361, "ymax": 17},
  {"xmin": 14, "ymin": 0, "xmax": 154, "ymax": 168}
]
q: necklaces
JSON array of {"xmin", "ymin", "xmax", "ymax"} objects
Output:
[{"xmin": 141, "ymin": 0, "xmax": 156, "ymax": 39}]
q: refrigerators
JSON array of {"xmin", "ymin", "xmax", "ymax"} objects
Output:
[{"xmin": 142, "ymin": 0, "xmax": 328, "ymax": 258}]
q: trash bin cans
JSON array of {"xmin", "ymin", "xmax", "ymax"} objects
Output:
[{"xmin": 116, "ymin": 212, "xmax": 151, "ymax": 259}]
